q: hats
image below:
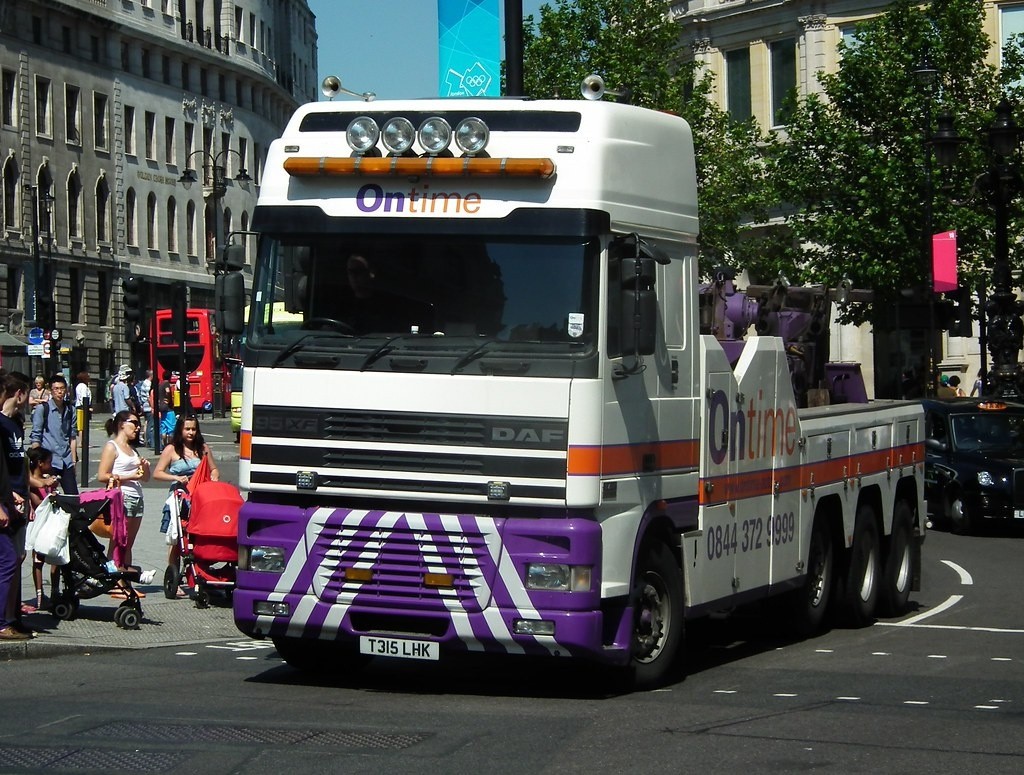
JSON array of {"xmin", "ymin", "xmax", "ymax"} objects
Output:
[
  {"xmin": 941, "ymin": 375, "xmax": 949, "ymax": 383},
  {"xmin": 118, "ymin": 364, "xmax": 132, "ymax": 373},
  {"xmin": 118, "ymin": 371, "xmax": 131, "ymax": 380}
]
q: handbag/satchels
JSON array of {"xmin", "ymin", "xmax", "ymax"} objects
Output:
[
  {"xmin": 166, "ymin": 494, "xmax": 178, "ymax": 545},
  {"xmin": 89, "ymin": 478, "xmax": 115, "ymax": 539},
  {"xmin": 25, "ymin": 493, "xmax": 70, "ymax": 565}
]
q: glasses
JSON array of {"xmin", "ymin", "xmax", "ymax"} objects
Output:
[{"xmin": 124, "ymin": 420, "xmax": 141, "ymax": 427}]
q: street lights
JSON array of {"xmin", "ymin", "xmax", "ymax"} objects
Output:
[{"xmin": 178, "ymin": 148, "xmax": 253, "ymax": 328}]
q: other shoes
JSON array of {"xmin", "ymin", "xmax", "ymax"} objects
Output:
[
  {"xmin": 37, "ymin": 596, "xmax": 45, "ymax": 609},
  {"xmin": 234, "ymin": 440, "xmax": 240, "ymax": 443},
  {"xmin": 0, "ymin": 628, "xmax": 30, "ymax": 641},
  {"xmin": 112, "ymin": 587, "xmax": 146, "ymax": 599},
  {"xmin": 50, "ymin": 593, "xmax": 61, "ymax": 603},
  {"xmin": 177, "ymin": 587, "xmax": 186, "ymax": 596}
]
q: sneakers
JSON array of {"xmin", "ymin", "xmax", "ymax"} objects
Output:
[
  {"xmin": 139, "ymin": 575, "xmax": 153, "ymax": 584},
  {"xmin": 142, "ymin": 569, "xmax": 156, "ymax": 575}
]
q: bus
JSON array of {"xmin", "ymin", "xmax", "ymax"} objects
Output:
[
  {"xmin": 227, "ymin": 301, "xmax": 305, "ymax": 440},
  {"xmin": 148, "ymin": 308, "xmax": 239, "ymax": 413}
]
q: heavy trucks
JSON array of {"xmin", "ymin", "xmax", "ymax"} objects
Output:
[{"xmin": 235, "ymin": 74, "xmax": 933, "ymax": 687}]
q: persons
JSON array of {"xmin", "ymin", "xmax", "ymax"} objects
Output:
[
  {"xmin": 939, "ymin": 368, "xmax": 987, "ymax": 400},
  {"xmin": 3, "ymin": 371, "xmax": 37, "ymax": 565},
  {"xmin": 0, "ymin": 368, "xmax": 30, "ymax": 641},
  {"xmin": 30, "ymin": 375, "xmax": 80, "ymax": 497},
  {"xmin": 98, "ymin": 409, "xmax": 151, "ymax": 598},
  {"xmin": 152, "ymin": 411, "xmax": 219, "ymax": 596},
  {"xmin": 25, "ymin": 445, "xmax": 65, "ymax": 610},
  {"xmin": 75, "ymin": 372, "xmax": 94, "ymax": 448},
  {"xmin": 901, "ymin": 367, "xmax": 925, "ymax": 400},
  {"xmin": 28, "ymin": 375, "xmax": 49, "ymax": 421},
  {"xmin": 106, "ymin": 365, "xmax": 194, "ymax": 450}
]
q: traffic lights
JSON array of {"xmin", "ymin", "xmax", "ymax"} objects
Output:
[{"xmin": 122, "ymin": 279, "xmax": 148, "ymax": 319}]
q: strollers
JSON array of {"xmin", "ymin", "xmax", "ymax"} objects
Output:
[
  {"xmin": 45, "ymin": 474, "xmax": 143, "ymax": 628},
  {"xmin": 163, "ymin": 471, "xmax": 245, "ymax": 607}
]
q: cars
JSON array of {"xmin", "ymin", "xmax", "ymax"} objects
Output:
[{"xmin": 914, "ymin": 397, "xmax": 1024, "ymax": 527}]
